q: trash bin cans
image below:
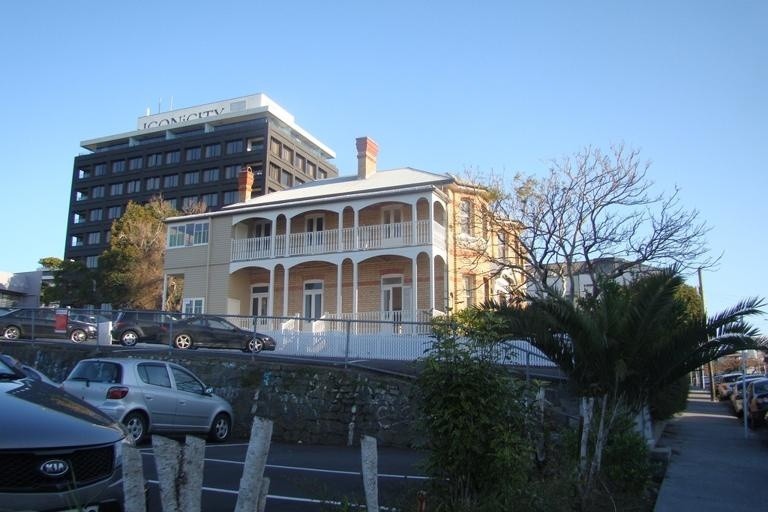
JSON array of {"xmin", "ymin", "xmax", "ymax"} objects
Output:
[
  {"xmin": 97, "ymin": 321, "xmax": 112, "ymax": 346},
  {"xmin": 55, "ymin": 310, "xmax": 70, "ymax": 333}
]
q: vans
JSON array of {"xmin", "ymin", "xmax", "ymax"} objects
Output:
[{"xmin": 112, "ymin": 311, "xmax": 184, "ymax": 347}]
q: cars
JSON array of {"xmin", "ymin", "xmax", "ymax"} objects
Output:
[
  {"xmin": 0, "ymin": 352, "xmax": 135, "ymax": 511},
  {"xmin": 60, "ymin": 356, "xmax": 235, "ymax": 445},
  {"xmin": 0, "ymin": 308, "xmax": 96, "ymax": 342},
  {"xmin": 693, "ymin": 373, "xmax": 768, "ymax": 430},
  {"xmin": 67, "ymin": 312, "xmax": 109, "ymax": 336},
  {"xmin": 157, "ymin": 315, "xmax": 276, "ymax": 354},
  {"xmin": 0, "ymin": 352, "xmax": 59, "ymax": 390}
]
